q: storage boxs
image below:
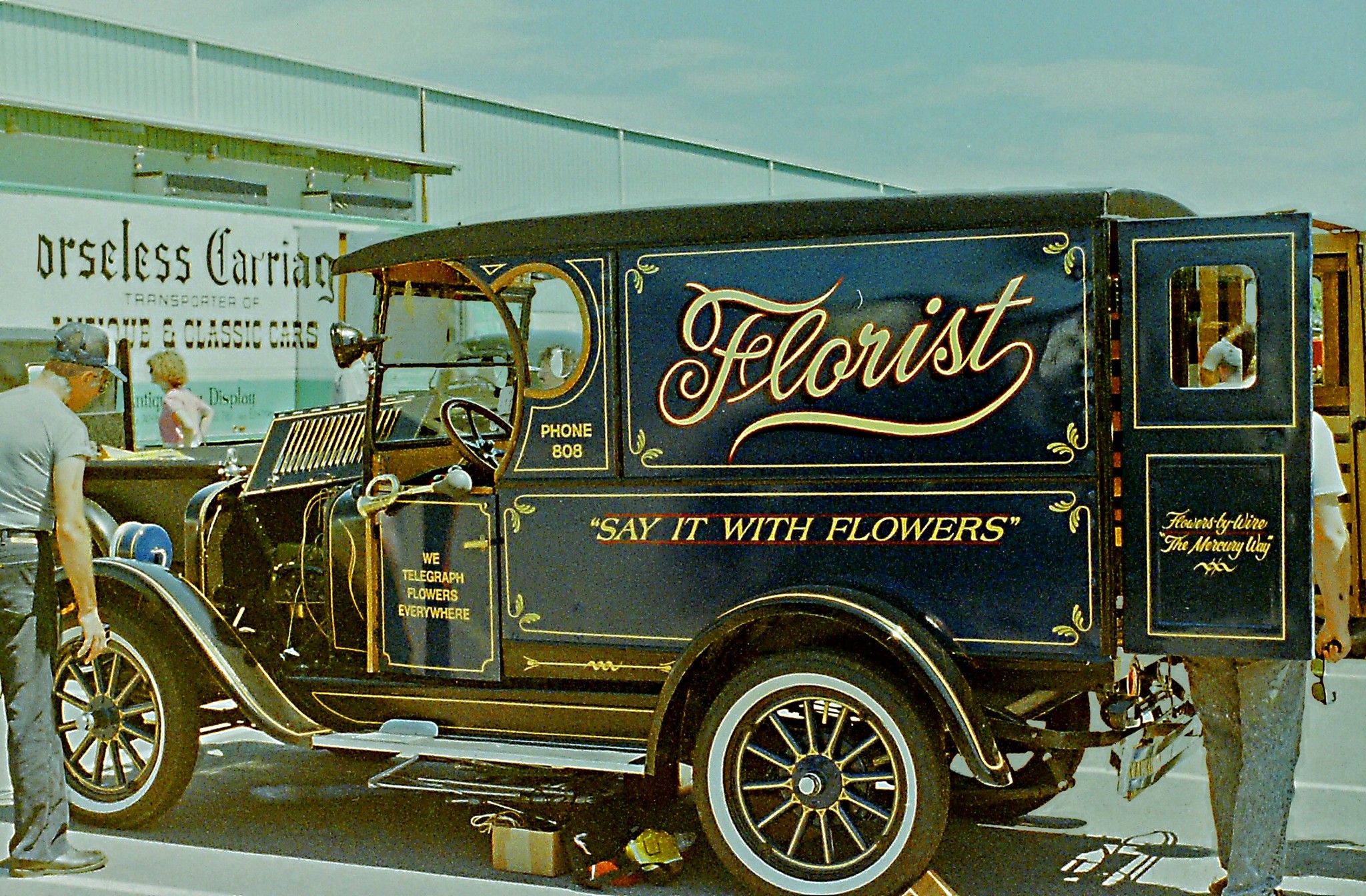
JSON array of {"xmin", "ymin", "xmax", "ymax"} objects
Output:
[{"xmin": 492, "ymin": 825, "xmax": 568, "ymax": 876}]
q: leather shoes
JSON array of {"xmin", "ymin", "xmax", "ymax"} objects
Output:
[{"xmin": 9, "ymin": 848, "xmax": 107, "ymax": 878}]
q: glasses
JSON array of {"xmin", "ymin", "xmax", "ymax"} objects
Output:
[{"xmin": 1310, "ymin": 647, "xmax": 1337, "ymax": 706}]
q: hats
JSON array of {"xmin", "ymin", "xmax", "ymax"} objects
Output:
[{"xmin": 49, "ymin": 321, "xmax": 128, "ymax": 383}]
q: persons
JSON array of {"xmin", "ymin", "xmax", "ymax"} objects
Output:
[
  {"xmin": 147, "ymin": 351, "xmax": 212, "ymax": 450},
  {"xmin": 1178, "ymin": 354, "xmax": 1351, "ymax": 895},
  {"xmin": 2, "ymin": 323, "xmax": 129, "ymax": 882}
]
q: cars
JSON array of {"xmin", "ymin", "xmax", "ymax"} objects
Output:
[{"xmin": 46, "ymin": 184, "xmax": 1365, "ymax": 896}]
